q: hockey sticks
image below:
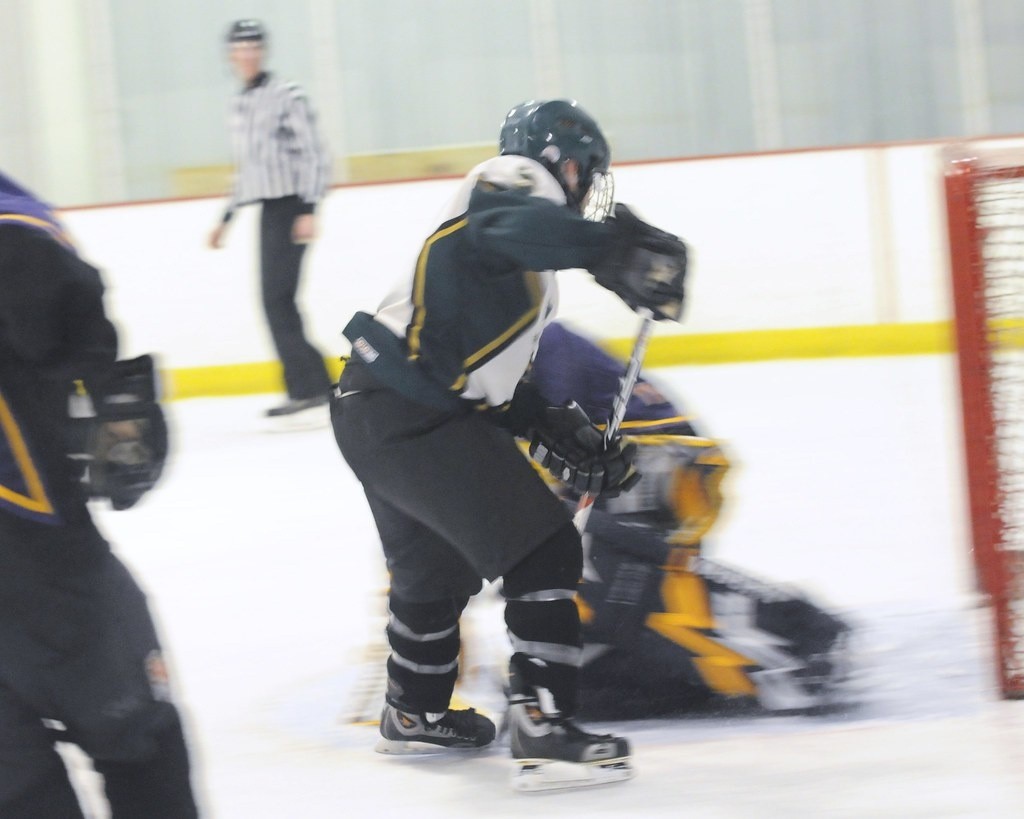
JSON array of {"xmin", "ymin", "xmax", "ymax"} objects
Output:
[{"xmin": 570, "ymin": 314, "xmax": 664, "ymax": 529}]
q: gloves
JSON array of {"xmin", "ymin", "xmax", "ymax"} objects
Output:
[
  {"xmin": 586, "ymin": 198, "xmax": 687, "ymax": 321},
  {"xmin": 528, "ymin": 399, "xmax": 643, "ymax": 499},
  {"xmin": 84, "ymin": 355, "xmax": 169, "ymax": 510}
]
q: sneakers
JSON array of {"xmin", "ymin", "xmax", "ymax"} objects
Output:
[
  {"xmin": 368, "ymin": 697, "xmax": 496, "ymax": 755},
  {"xmin": 264, "ymin": 390, "xmax": 333, "ymax": 431},
  {"xmin": 508, "ymin": 652, "xmax": 635, "ymax": 793}
]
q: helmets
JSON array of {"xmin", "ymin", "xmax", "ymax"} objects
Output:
[
  {"xmin": 499, "ymin": 99, "xmax": 615, "ymax": 222},
  {"xmin": 221, "ymin": 19, "xmax": 266, "ymax": 44}
]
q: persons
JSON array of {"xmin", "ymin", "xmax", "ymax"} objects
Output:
[
  {"xmin": 518, "ymin": 273, "xmax": 849, "ymax": 720},
  {"xmin": 332, "ymin": 101, "xmax": 685, "ymax": 794},
  {"xmin": 0, "ymin": 175, "xmax": 200, "ymax": 818},
  {"xmin": 207, "ymin": 18, "xmax": 335, "ymax": 417}
]
